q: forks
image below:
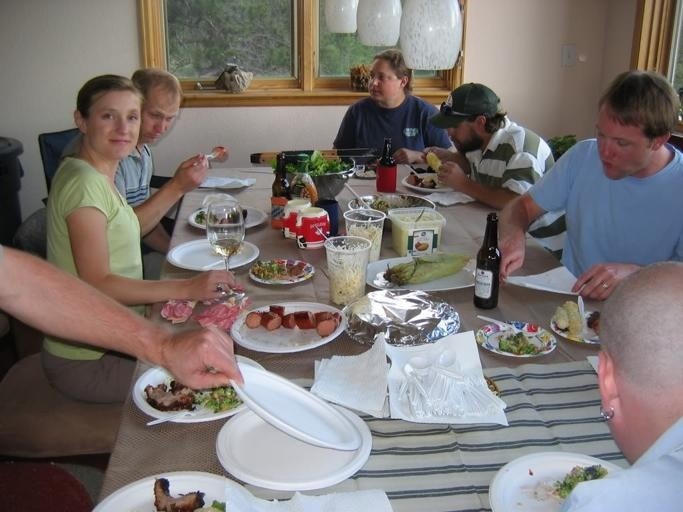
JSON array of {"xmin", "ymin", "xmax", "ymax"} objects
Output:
[
  {"xmin": 189, "ymin": 150, "xmax": 221, "ymax": 168},
  {"xmin": 143, "ymin": 404, "xmax": 217, "ymax": 426}
]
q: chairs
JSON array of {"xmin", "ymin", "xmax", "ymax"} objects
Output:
[
  {"xmin": 16, "ymin": 207, "xmax": 164, "ymax": 291},
  {"xmin": 38, "ymin": 127, "xmax": 179, "ymax": 244},
  {"xmin": 0, "ymin": 312, "xmax": 122, "ymax": 459}
]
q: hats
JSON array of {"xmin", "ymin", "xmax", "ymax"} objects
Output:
[{"xmin": 427, "ymin": 83, "xmax": 500, "ymax": 128}]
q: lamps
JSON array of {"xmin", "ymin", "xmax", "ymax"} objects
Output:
[
  {"xmin": 399, "ymin": 0, "xmax": 463, "ymax": 71},
  {"xmin": 356, "ymin": 0, "xmax": 402, "ymax": 48},
  {"xmin": 323, "ymin": 1, "xmax": 358, "ymax": 34}
]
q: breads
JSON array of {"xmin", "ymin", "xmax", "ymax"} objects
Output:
[
  {"xmin": 555, "ymin": 307, "xmax": 569, "ymax": 330},
  {"xmin": 562, "ymin": 301, "xmax": 581, "ymax": 335}
]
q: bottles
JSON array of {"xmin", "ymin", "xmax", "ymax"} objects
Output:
[
  {"xmin": 271, "ymin": 151, "xmax": 290, "ymax": 200},
  {"xmin": 373, "ymin": 137, "xmax": 397, "ymax": 193},
  {"xmin": 469, "ymin": 211, "xmax": 502, "ymax": 311}
]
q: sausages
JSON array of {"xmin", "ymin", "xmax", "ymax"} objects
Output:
[{"xmin": 246, "ymin": 304, "xmax": 341, "ymax": 336}]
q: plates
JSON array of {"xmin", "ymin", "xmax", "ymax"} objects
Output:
[
  {"xmin": 401, "ymin": 171, "xmax": 453, "ymax": 193},
  {"xmin": 130, "ymin": 353, "xmax": 265, "ymax": 424},
  {"xmin": 187, "ymin": 206, "xmax": 269, "ymax": 232},
  {"xmin": 229, "ymin": 300, "xmax": 347, "ymax": 357},
  {"xmin": 551, "ymin": 307, "xmax": 601, "ymax": 345},
  {"xmin": 165, "ymin": 236, "xmax": 257, "ymax": 272},
  {"xmin": 245, "ymin": 258, "xmax": 316, "ymax": 287},
  {"xmin": 226, "ymin": 362, "xmax": 363, "ymax": 452},
  {"xmin": 214, "ymin": 399, "xmax": 372, "ymax": 491},
  {"xmin": 485, "ymin": 449, "xmax": 625, "ymax": 511},
  {"xmin": 475, "ymin": 317, "xmax": 555, "ymax": 359},
  {"xmin": 90, "ymin": 470, "xmax": 258, "ymax": 512},
  {"xmin": 364, "ymin": 254, "xmax": 479, "ymax": 295}
]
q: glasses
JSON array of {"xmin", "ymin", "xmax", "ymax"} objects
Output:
[{"xmin": 440, "ymin": 102, "xmax": 471, "ymax": 117}]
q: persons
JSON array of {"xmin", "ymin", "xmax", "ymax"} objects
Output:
[
  {"xmin": 497, "ymin": 71, "xmax": 683, "ymax": 300},
  {"xmin": 422, "ymin": 82, "xmax": 568, "ymax": 262},
  {"xmin": 556, "ymin": 261, "xmax": 683, "ymax": 511},
  {"xmin": 42, "ymin": 74, "xmax": 239, "ymax": 405},
  {"xmin": 0, "ymin": 245, "xmax": 244, "ymax": 512},
  {"xmin": 335, "ymin": 48, "xmax": 455, "ymax": 164},
  {"xmin": 61, "ymin": 68, "xmax": 209, "ymax": 282}
]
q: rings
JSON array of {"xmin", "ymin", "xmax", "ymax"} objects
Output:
[
  {"xmin": 602, "ymin": 281, "xmax": 608, "ymax": 289},
  {"xmin": 216, "ymin": 282, "xmax": 223, "ymax": 291}
]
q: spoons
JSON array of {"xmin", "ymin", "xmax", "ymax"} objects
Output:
[
  {"xmin": 397, "ymin": 349, "xmax": 507, "ymax": 420},
  {"xmin": 471, "ymin": 312, "xmax": 512, "ymax": 332}
]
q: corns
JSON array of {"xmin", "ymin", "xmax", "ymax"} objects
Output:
[
  {"xmin": 384, "ymin": 254, "xmax": 468, "ymax": 283},
  {"xmin": 425, "ymin": 151, "xmax": 442, "ymax": 173}
]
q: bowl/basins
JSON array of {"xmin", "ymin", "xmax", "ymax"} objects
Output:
[
  {"xmin": 291, "ymin": 158, "xmax": 356, "ymax": 200},
  {"xmin": 348, "ymin": 193, "xmax": 436, "ymax": 228}
]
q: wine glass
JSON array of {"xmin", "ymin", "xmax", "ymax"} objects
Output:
[{"xmin": 206, "ymin": 199, "xmax": 245, "ymax": 300}]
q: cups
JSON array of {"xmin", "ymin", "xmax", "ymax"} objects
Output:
[
  {"xmin": 342, "ymin": 210, "xmax": 385, "ymax": 262},
  {"xmin": 321, "ymin": 235, "xmax": 370, "ymax": 305}
]
